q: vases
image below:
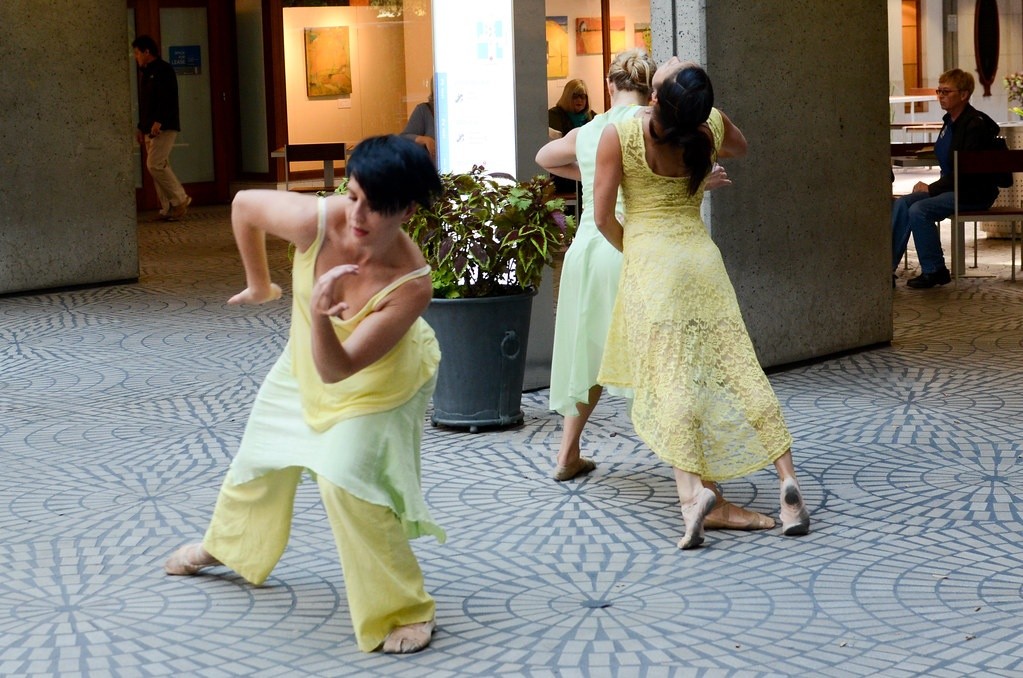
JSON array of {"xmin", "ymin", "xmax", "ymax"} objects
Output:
[{"xmin": 428, "ymin": 285, "xmax": 539, "ymax": 431}]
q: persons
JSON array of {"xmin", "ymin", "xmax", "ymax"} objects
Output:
[
  {"xmin": 891, "ymin": 68, "xmax": 999, "ymax": 289},
  {"xmin": 164, "ymin": 133, "xmax": 447, "ymax": 654},
  {"xmin": 535, "ymin": 48, "xmax": 810, "ymax": 550},
  {"xmin": 399, "ymin": 75, "xmax": 437, "ymax": 156},
  {"xmin": 131, "ymin": 35, "xmax": 192, "ymax": 222}
]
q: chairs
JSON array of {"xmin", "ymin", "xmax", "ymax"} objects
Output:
[{"xmin": 937, "ymin": 149, "xmax": 1023, "ymax": 283}]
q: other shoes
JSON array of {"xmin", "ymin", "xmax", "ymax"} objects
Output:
[
  {"xmin": 676, "ymin": 487, "xmax": 717, "ymax": 549},
  {"xmin": 905, "ymin": 269, "xmax": 950, "ymax": 289},
  {"xmin": 164, "ymin": 543, "xmax": 224, "ymax": 575},
  {"xmin": 382, "ymin": 619, "xmax": 436, "ymax": 653},
  {"xmin": 170, "ymin": 196, "xmax": 192, "ymax": 217},
  {"xmin": 780, "ymin": 479, "xmax": 810, "ymax": 536},
  {"xmin": 554, "ymin": 458, "xmax": 596, "ymax": 480},
  {"xmin": 705, "ymin": 501, "xmax": 782, "ymax": 530}
]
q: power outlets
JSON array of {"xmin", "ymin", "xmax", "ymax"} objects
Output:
[{"xmin": 338, "ymin": 98, "xmax": 351, "ymax": 109}]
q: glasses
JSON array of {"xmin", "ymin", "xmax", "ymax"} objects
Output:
[
  {"xmin": 935, "ymin": 89, "xmax": 958, "ymax": 95},
  {"xmin": 572, "ymin": 93, "xmax": 587, "ymax": 100}
]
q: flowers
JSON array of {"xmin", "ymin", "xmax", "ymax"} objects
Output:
[{"xmin": 335, "ymin": 165, "xmax": 577, "ymax": 298}]
